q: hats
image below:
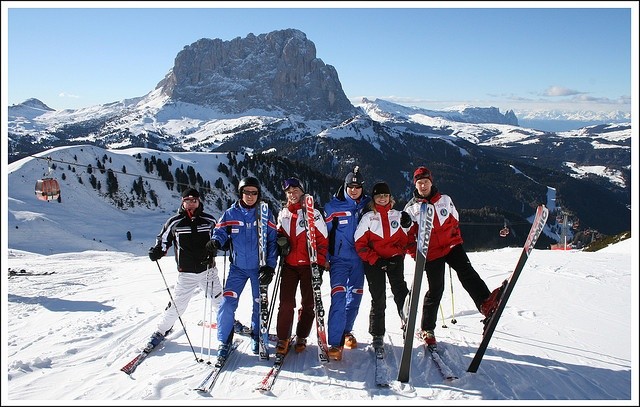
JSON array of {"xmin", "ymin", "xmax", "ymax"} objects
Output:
[
  {"xmin": 414, "ymin": 166, "xmax": 432, "ymax": 184},
  {"xmin": 183, "ymin": 188, "xmax": 199, "ymax": 197},
  {"xmin": 345, "ymin": 171, "xmax": 363, "ymax": 183},
  {"xmin": 373, "ymin": 183, "xmax": 390, "ymax": 194}
]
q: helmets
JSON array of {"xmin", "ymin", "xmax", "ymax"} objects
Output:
[
  {"xmin": 238, "ymin": 177, "xmax": 261, "ymax": 198},
  {"xmin": 282, "ymin": 176, "xmax": 305, "ymax": 193}
]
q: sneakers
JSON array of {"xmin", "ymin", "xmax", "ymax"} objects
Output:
[
  {"xmin": 343, "ymin": 334, "xmax": 357, "ymax": 349},
  {"xmin": 480, "ymin": 287, "xmax": 503, "ymax": 318},
  {"xmin": 151, "ymin": 328, "xmax": 167, "ymax": 346},
  {"xmin": 418, "ymin": 329, "xmax": 436, "ymax": 345},
  {"xmin": 276, "ymin": 339, "xmax": 289, "ymax": 354},
  {"xmin": 329, "ymin": 346, "xmax": 342, "ymax": 360},
  {"xmin": 373, "ymin": 336, "xmax": 384, "ymax": 348},
  {"xmin": 217, "ymin": 344, "xmax": 230, "ymax": 360},
  {"xmin": 402, "ymin": 292, "xmax": 411, "ymax": 327},
  {"xmin": 251, "ymin": 337, "xmax": 259, "ymax": 354},
  {"xmin": 233, "ymin": 320, "xmax": 248, "ymax": 331},
  {"xmin": 296, "ymin": 337, "xmax": 306, "ymax": 352}
]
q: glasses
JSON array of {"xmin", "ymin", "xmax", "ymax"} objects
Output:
[
  {"xmin": 183, "ymin": 199, "xmax": 199, "ymax": 203},
  {"xmin": 347, "ymin": 184, "xmax": 362, "ymax": 189},
  {"xmin": 242, "ymin": 190, "xmax": 259, "ymax": 195},
  {"xmin": 282, "ymin": 178, "xmax": 299, "ymax": 191},
  {"xmin": 376, "ymin": 191, "xmax": 389, "ymax": 196},
  {"xmin": 415, "ymin": 173, "xmax": 431, "ymax": 179}
]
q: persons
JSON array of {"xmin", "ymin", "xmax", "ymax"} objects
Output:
[
  {"xmin": 207, "ymin": 176, "xmax": 278, "ymax": 366},
  {"xmin": 354, "ymin": 181, "xmax": 411, "ymax": 356},
  {"xmin": 323, "ymin": 171, "xmax": 372, "ymax": 360},
  {"xmin": 276, "ymin": 176, "xmax": 329, "ymax": 354},
  {"xmin": 404, "ymin": 166, "xmax": 506, "ymax": 350},
  {"xmin": 143, "ymin": 186, "xmax": 251, "ymax": 354}
]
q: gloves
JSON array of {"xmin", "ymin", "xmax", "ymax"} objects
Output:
[
  {"xmin": 400, "ymin": 211, "xmax": 412, "ymax": 228},
  {"xmin": 201, "ymin": 253, "xmax": 213, "ymax": 265},
  {"xmin": 257, "ymin": 265, "xmax": 274, "ymax": 285},
  {"xmin": 148, "ymin": 246, "xmax": 162, "ymax": 261},
  {"xmin": 205, "ymin": 240, "xmax": 219, "ymax": 252},
  {"xmin": 378, "ymin": 260, "xmax": 396, "ymax": 271},
  {"xmin": 277, "ymin": 236, "xmax": 290, "ymax": 248}
]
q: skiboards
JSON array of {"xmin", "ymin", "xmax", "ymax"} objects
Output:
[
  {"xmin": 120, "ymin": 320, "xmax": 294, "ymax": 373},
  {"xmin": 256, "ymin": 193, "xmax": 326, "ymax": 391},
  {"xmin": 372, "ymin": 204, "xmax": 434, "ymax": 388},
  {"xmin": 193, "ymin": 200, "xmax": 269, "ymax": 394},
  {"xmin": 416, "ymin": 205, "xmax": 547, "ymax": 382}
]
